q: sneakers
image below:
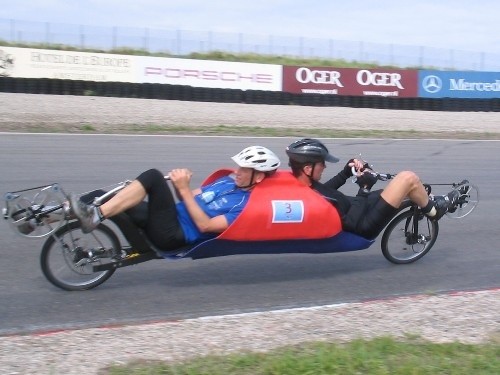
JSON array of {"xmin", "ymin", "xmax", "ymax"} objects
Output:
[
  {"xmin": 5, "ymin": 192, "xmax": 39, "ymax": 236},
  {"xmin": 424, "ymin": 190, "xmax": 461, "ymax": 223},
  {"xmin": 68, "ymin": 191, "xmax": 101, "ymax": 234},
  {"xmin": 399, "ymin": 183, "xmax": 433, "ymax": 210}
]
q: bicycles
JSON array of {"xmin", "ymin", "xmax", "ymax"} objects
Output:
[{"xmin": 2, "ymin": 154, "xmax": 481, "ymax": 292}]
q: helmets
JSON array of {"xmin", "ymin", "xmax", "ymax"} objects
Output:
[
  {"xmin": 230, "ymin": 145, "xmax": 281, "ymax": 172},
  {"xmin": 285, "ymin": 137, "xmax": 340, "ymax": 164}
]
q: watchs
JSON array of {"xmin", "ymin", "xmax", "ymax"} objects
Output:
[{"xmin": 360, "ymin": 188, "xmax": 370, "ymax": 193}]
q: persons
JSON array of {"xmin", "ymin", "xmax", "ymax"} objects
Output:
[
  {"xmin": 286, "ymin": 138, "xmax": 469, "ymax": 240},
  {"xmin": 2, "ymin": 145, "xmax": 281, "ymax": 252}
]
